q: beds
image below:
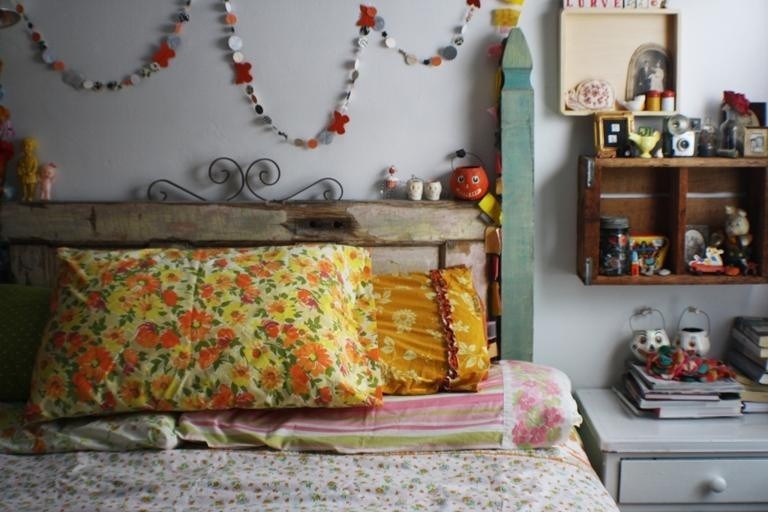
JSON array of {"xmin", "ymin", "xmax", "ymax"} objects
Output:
[{"xmin": 0, "ymin": 198, "xmax": 621, "ymax": 512}]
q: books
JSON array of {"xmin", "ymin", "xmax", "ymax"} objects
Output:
[{"xmin": 610, "ymin": 315, "xmax": 768, "ymax": 420}]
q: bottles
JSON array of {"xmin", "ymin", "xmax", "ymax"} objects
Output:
[
  {"xmin": 701, "ymin": 116, "xmax": 716, "ymax": 155},
  {"xmin": 720, "ymin": 111, "xmax": 744, "ymax": 158},
  {"xmin": 646, "ymin": 91, "xmax": 674, "ymax": 112},
  {"xmin": 599, "ymin": 216, "xmax": 630, "ymax": 277}
]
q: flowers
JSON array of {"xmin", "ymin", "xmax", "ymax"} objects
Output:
[{"xmin": 723, "ymin": 91, "xmax": 753, "ymax": 119}]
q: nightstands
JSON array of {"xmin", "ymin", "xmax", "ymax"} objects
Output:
[{"xmin": 575, "ymin": 388, "xmax": 768, "ymax": 512}]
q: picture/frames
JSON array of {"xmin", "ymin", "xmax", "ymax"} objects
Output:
[
  {"xmin": 594, "ymin": 111, "xmax": 636, "ymax": 157},
  {"xmin": 745, "ymin": 126, "xmax": 768, "ymax": 157}
]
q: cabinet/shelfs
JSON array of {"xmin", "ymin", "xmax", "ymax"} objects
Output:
[
  {"xmin": 558, "ymin": 9, "xmax": 680, "ymax": 117},
  {"xmin": 576, "ymin": 157, "xmax": 768, "ymax": 286}
]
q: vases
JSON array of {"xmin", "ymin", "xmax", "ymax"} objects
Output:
[{"xmin": 716, "ymin": 107, "xmax": 745, "ymax": 156}]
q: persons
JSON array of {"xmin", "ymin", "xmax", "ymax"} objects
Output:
[
  {"xmin": 751, "ymin": 136, "xmax": 763, "ymax": 152},
  {"xmin": 0, "ymin": 90, "xmax": 16, "ymax": 197},
  {"xmin": 638, "ymin": 60, "xmax": 664, "ymax": 92},
  {"xmin": 15, "ymin": 136, "xmax": 39, "ymax": 201},
  {"xmin": 640, "ymin": 242, "xmax": 648, "ymax": 254},
  {"xmin": 37, "ymin": 162, "xmax": 56, "ymax": 201}
]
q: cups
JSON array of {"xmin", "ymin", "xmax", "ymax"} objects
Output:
[{"xmin": 451, "ymin": 165, "xmax": 490, "ymax": 201}]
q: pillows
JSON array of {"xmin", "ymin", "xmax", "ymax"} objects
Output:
[
  {"xmin": 25, "ymin": 241, "xmax": 383, "ymax": 414},
  {"xmin": 373, "ymin": 263, "xmax": 493, "ymax": 396},
  {"xmin": 176, "ymin": 356, "xmax": 582, "ymax": 451},
  {"xmin": 0, "ymin": 398, "xmax": 180, "ymax": 455}
]
